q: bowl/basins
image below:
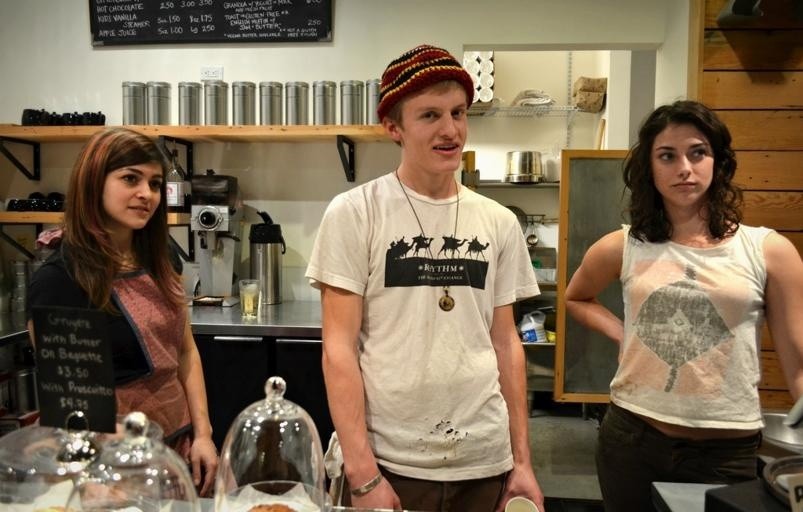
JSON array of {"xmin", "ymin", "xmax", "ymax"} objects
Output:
[{"xmin": 503, "ymin": 151, "xmax": 545, "ymax": 184}]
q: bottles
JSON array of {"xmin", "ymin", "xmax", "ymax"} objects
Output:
[
  {"xmin": 120, "ymin": 77, "xmax": 383, "ymax": 126},
  {"xmin": 165, "ymin": 149, "xmax": 189, "ymax": 213},
  {"xmin": 247, "ymin": 209, "xmax": 286, "ymax": 305}
]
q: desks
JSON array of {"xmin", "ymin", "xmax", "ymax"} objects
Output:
[{"xmin": 650, "ymin": 476, "xmax": 790, "ymax": 512}]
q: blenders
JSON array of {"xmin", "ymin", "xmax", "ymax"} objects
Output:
[{"xmin": 185, "ymin": 169, "xmax": 246, "ymax": 309}]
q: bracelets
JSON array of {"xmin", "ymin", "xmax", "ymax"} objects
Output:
[{"xmin": 351, "ymin": 472, "xmax": 382, "ymax": 495}]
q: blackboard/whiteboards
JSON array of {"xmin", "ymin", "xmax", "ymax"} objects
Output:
[{"xmin": 89, "ymin": 0, "xmax": 338, "ymax": 50}]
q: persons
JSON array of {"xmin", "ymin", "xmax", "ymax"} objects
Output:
[
  {"xmin": 562, "ymin": 101, "xmax": 802, "ymax": 511},
  {"xmin": 26, "ymin": 125, "xmax": 218, "ymax": 512},
  {"xmin": 304, "ymin": 44, "xmax": 546, "ymax": 511}
]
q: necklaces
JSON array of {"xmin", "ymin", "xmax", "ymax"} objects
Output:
[{"xmin": 395, "ymin": 168, "xmax": 458, "ymax": 311}]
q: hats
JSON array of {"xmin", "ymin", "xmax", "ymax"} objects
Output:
[{"xmin": 377, "ymin": 46, "xmax": 474, "ymax": 122}]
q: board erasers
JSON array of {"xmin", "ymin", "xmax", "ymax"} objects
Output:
[{"xmin": 93, "ymin": 40, "xmax": 104, "ymax": 45}]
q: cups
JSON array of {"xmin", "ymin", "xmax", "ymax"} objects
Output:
[
  {"xmin": 237, "ymin": 277, "xmax": 261, "ymax": 319},
  {"xmin": 7, "ymin": 191, "xmax": 65, "ymax": 211}
]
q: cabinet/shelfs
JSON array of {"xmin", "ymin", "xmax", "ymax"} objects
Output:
[
  {"xmin": 192, "ymin": 335, "xmax": 345, "ymax": 457},
  {"xmin": 466, "ymin": 102, "xmax": 604, "ymax": 417},
  {"xmin": 0, "ymin": 121, "xmax": 395, "ymax": 264}
]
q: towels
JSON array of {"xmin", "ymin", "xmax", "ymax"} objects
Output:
[{"xmin": 508, "ymin": 89, "xmax": 555, "ymax": 115}]
